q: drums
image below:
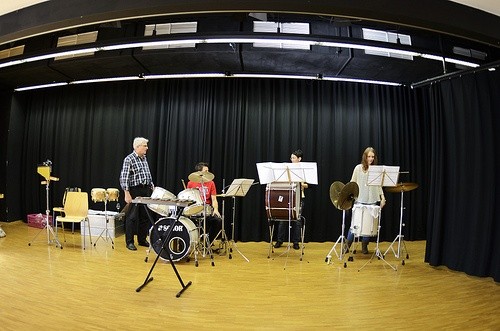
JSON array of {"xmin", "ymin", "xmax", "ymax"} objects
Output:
[
  {"xmin": 148, "ymin": 215, "xmax": 199, "ymax": 262},
  {"xmin": 177, "ymin": 187, "xmax": 204, "ymax": 216},
  {"xmin": 92, "ymin": 188, "xmax": 106, "ymax": 202},
  {"xmin": 107, "ymin": 188, "xmax": 119, "ymax": 200},
  {"xmin": 146, "ymin": 186, "xmax": 177, "ymax": 216},
  {"xmin": 265, "ymin": 182, "xmax": 301, "ymax": 220}
]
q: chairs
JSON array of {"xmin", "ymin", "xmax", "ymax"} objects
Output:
[
  {"xmin": 52, "ymin": 188, "xmax": 81, "ymax": 234},
  {"xmin": 54, "ymin": 192, "xmax": 92, "ymax": 250}
]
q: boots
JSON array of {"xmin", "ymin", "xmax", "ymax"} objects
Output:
[
  {"xmin": 343, "ymin": 239, "xmax": 352, "ymax": 254},
  {"xmin": 362, "ymin": 240, "xmax": 368, "ymax": 254}
]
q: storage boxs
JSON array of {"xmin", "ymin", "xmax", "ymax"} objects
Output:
[
  {"xmin": 80, "ymin": 209, "xmax": 125, "ymax": 238},
  {"xmin": 28, "ymin": 214, "xmax": 53, "ymax": 229}
]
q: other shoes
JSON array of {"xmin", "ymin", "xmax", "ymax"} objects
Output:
[
  {"xmin": 294, "ymin": 243, "xmax": 299, "ymax": 249},
  {"xmin": 206, "ymin": 241, "xmax": 220, "ymax": 253},
  {"xmin": 143, "ymin": 240, "xmax": 149, "ymax": 246},
  {"xmin": 126, "ymin": 243, "xmax": 137, "ymax": 250},
  {"xmin": 274, "ymin": 241, "xmax": 283, "ymax": 248}
]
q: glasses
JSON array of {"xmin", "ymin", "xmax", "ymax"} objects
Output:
[{"xmin": 290, "ymin": 157, "xmax": 296, "ymax": 160}]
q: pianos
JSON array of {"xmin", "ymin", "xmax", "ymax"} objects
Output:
[{"xmin": 129, "ymin": 196, "xmax": 197, "ymax": 299}]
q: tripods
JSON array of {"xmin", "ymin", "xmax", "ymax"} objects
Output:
[
  {"xmin": 184, "ymin": 175, "xmax": 254, "ymax": 267},
  {"xmin": 325, "ymin": 209, "xmax": 354, "ymax": 268},
  {"xmin": 356, "ymin": 165, "xmax": 399, "ymax": 272},
  {"xmin": 27, "ymin": 163, "xmax": 62, "ymax": 249},
  {"xmin": 381, "ymin": 190, "xmax": 410, "ymax": 267},
  {"xmin": 93, "ymin": 195, "xmax": 114, "ymax": 250}
]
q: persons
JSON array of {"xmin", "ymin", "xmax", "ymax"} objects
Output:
[
  {"xmin": 342, "ymin": 147, "xmax": 385, "ymax": 254},
  {"xmin": 120, "ymin": 137, "xmax": 154, "ymax": 250},
  {"xmin": 186, "ymin": 162, "xmax": 223, "ymax": 248},
  {"xmin": 274, "ymin": 150, "xmax": 311, "ymax": 249}
]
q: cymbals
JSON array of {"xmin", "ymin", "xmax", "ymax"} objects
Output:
[
  {"xmin": 188, "ymin": 171, "xmax": 215, "ymax": 183},
  {"xmin": 213, "ymin": 193, "xmax": 225, "ymax": 197},
  {"xmin": 386, "ymin": 182, "xmax": 418, "ymax": 192},
  {"xmin": 329, "ymin": 181, "xmax": 359, "ymax": 210}
]
